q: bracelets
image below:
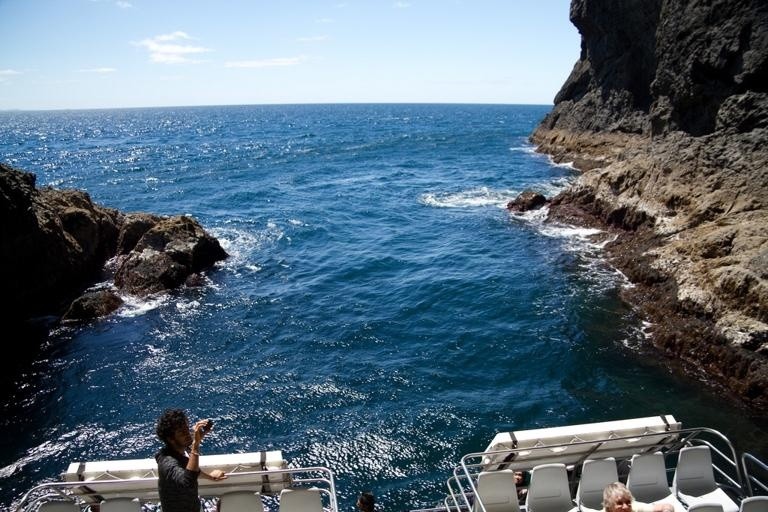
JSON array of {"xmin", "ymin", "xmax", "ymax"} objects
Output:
[{"xmin": 190, "ymin": 451, "xmax": 199, "ymax": 456}]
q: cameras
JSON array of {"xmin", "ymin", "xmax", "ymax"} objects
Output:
[{"xmin": 200, "ymin": 419, "xmax": 214, "ymax": 433}]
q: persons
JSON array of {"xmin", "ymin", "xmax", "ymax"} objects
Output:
[
  {"xmin": 155, "ymin": 408, "xmax": 227, "ymax": 512},
  {"xmin": 356, "ymin": 493, "xmax": 384, "ymax": 512},
  {"xmin": 600, "ymin": 481, "xmax": 674, "ymax": 512},
  {"xmin": 512, "ymin": 470, "xmax": 530, "ymax": 502}
]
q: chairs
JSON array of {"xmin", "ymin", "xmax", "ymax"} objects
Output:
[
  {"xmin": 473, "ymin": 445, "xmax": 768, "ymax": 512},
  {"xmin": 36, "ymin": 483, "xmax": 325, "ymax": 512}
]
